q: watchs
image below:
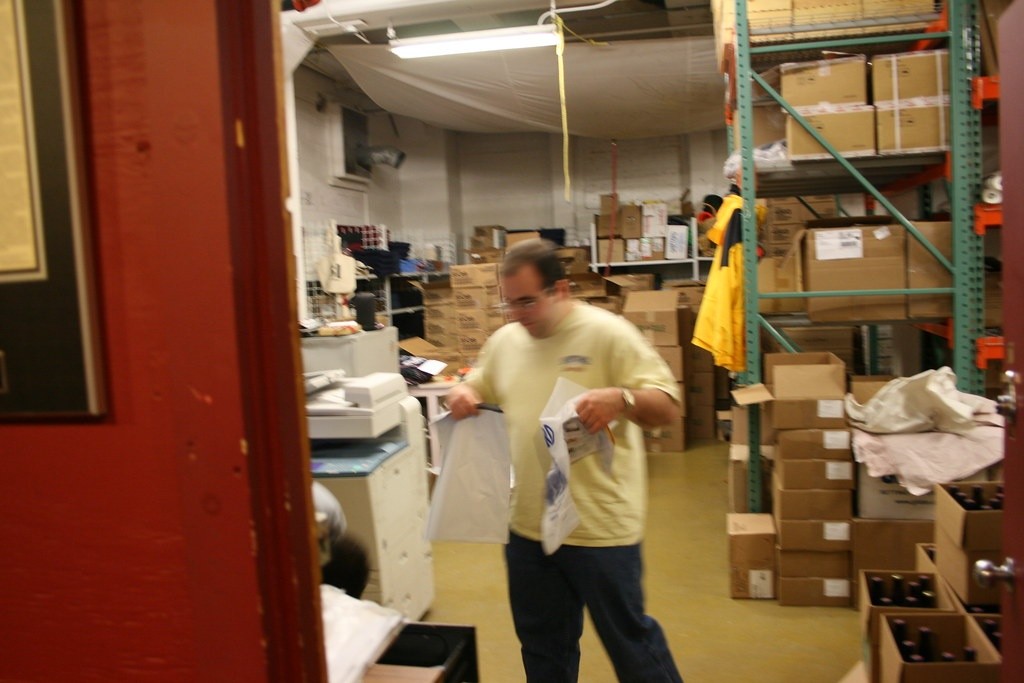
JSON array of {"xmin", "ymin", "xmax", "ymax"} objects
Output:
[{"xmin": 618, "ymin": 386, "xmax": 637, "ymax": 418}]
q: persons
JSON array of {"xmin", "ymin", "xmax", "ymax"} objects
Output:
[
  {"xmin": 340, "ymin": 232, "xmax": 388, "ymax": 331},
  {"xmin": 446, "ymin": 237, "xmax": 684, "ymax": 682}
]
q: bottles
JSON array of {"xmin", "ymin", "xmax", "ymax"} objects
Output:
[{"xmin": 868, "ymin": 483, "xmax": 1002, "ymax": 662}]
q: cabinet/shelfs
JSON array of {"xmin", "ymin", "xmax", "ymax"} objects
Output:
[{"xmin": 726, "ymin": 0, "xmax": 1000, "ymax": 512}]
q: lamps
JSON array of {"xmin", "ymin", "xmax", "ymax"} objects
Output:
[{"xmin": 385, "ymin": 24, "xmax": 560, "ymax": 59}]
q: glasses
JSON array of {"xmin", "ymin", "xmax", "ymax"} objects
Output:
[{"xmin": 496, "ymin": 288, "xmax": 554, "ymax": 314}]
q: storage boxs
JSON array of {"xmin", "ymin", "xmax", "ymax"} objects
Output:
[{"xmin": 395, "ymin": 0, "xmax": 1024, "ymax": 683}]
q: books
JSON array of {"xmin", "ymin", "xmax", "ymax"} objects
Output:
[{"xmin": 319, "ymin": 584, "xmax": 407, "ymax": 683}]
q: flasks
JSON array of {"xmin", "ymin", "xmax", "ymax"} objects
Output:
[{"xmin": 356, "ymin": 291, "xmax": 376, "ymax": 331}]
